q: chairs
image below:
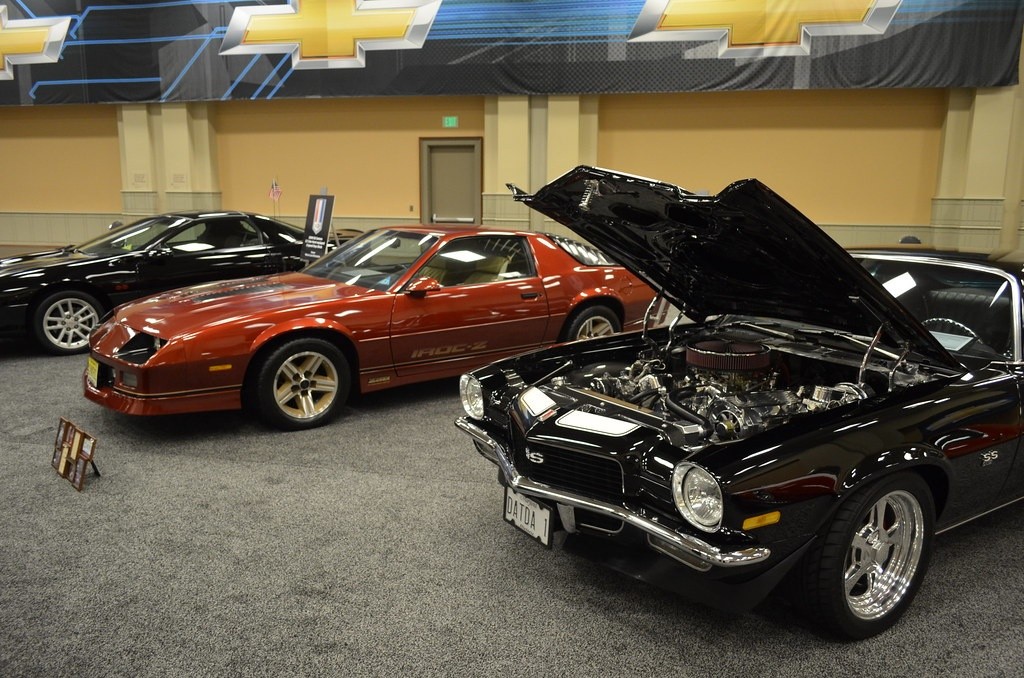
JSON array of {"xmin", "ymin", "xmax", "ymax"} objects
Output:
[
  {"xmin": 921, "ymin": 292, "xmax": 1014, "ymax": 355},
  {"xmin": 462, "ymin": 254, "xmax": 510, "ymax": 285},
  {"xmin": 417, "ymin": 241, "xmax": 457, "ymax": 287}
]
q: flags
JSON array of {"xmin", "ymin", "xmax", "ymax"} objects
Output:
[{"xmin": 270, "ymin": 180, "xmax": 280, "ymax": 202}]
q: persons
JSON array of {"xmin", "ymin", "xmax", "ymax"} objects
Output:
[{"xmin": 899, "ymin": 236, "xmax": 921, "ymax": 244}]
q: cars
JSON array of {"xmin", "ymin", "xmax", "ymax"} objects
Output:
[
  {"xmin": 453, "ymin": 160, "xmax": 1023, "ymax": 642},
  {"xmin": 82, "ymin": 224, "xmax": 671, "ymax": 435},
  {"xmin": 0, "ymin": 210, "xmax": 364, "ymax": 357}
]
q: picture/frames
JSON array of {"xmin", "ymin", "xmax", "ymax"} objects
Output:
[{"xmin": 50, "ymin": 418, "xmax": 99, "ymax": 492}]
